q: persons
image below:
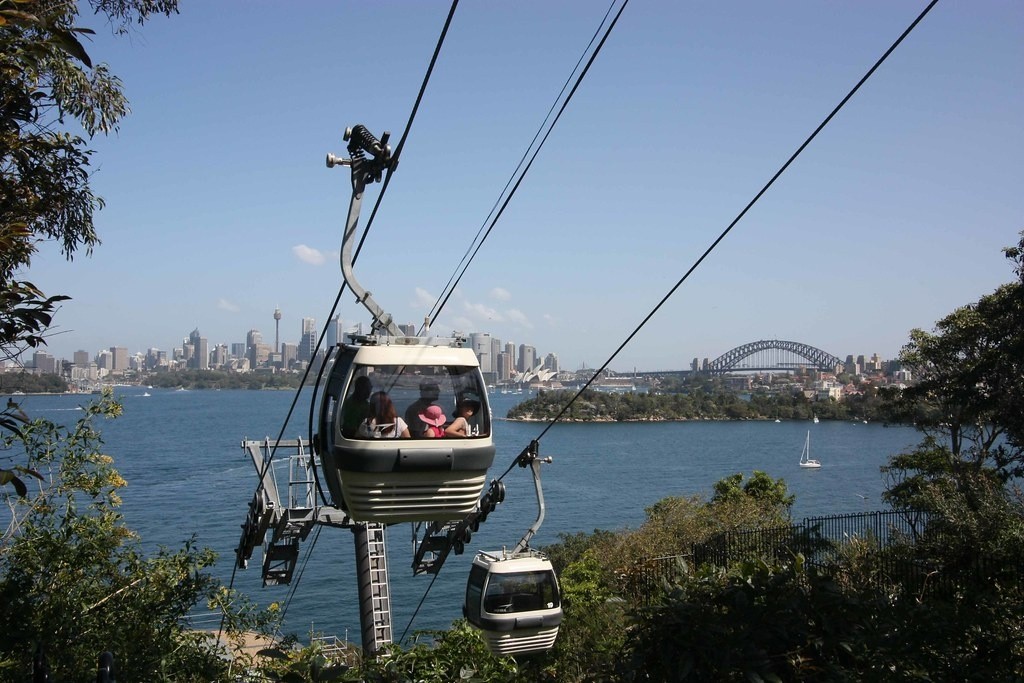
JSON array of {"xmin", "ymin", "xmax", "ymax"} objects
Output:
[
  {"xmin": 405, "ymin": 378, "xmax": 440, "ymax": 434},
  {"xmin": 444, "ymin": 393, "xmax": 481, "ymax": 437},
  {"xmin": 356, "ymin": 391, "xmax": 411, "ymax": 438},
  {"xmin": 417, "ymin": 406, "xmax": 447, "ymax": 437},
  {"xmin": 343, "ymin": 376, "xmax": 372, "ymax": 438}
]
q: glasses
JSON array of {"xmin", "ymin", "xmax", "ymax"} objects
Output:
[{"xmin": 364, "ymin": 386, "xmax": 371, "ymax": 388}]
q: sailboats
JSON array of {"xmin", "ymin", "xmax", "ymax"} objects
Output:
[{"xmin": 799, "ymin": 430, "xmax": 821, "ymax": 467}]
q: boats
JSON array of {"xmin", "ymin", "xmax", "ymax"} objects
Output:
[
  {"xmin": 813, "ymin": 416, "xmax": 819, "ymax": 423},
  {"xmin": 142, "ymin": 391, "xmax": 151, "ymax": 397},
  {"xmin": 488, "ymin": 380, "xmax": 661, "ymax": 397},
  {"xmin": 147, "ymin": 385, "xmax": 153, "ymax": 389},
  {"xmin": 775, "ymin": 419, "xmax": 781, "ymax": 422},
  {"xmin": 12, "ymin": 390, "xmax": 25, "ymax": 396}
]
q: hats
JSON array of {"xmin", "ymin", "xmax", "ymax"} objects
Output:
[
  {"xmin": 417, "ymin": 404, "xmax": 447, "ymax": 428},
  {"xmin": 452, "ymin": 392, "xmax": 481, "ymax": 418}
]
